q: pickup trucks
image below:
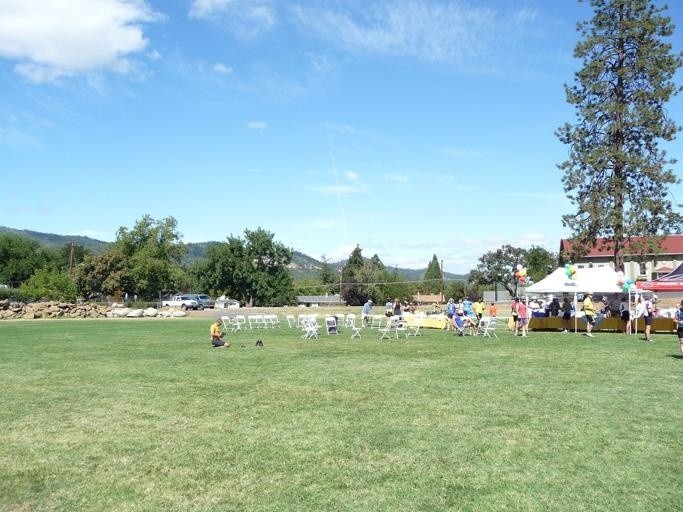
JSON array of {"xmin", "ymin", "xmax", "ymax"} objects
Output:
[{"xmin": 162, "ymin": 294, "xmax": 199, "ymax": 311}]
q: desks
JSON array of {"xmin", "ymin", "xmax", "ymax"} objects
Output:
[
  {"xmin": 404, "ymin": 313, "xmax": 447, "ymax": 330},
  {"xmin": 527, "ymin": 317, "xmax": 675, "ymax": 334}
]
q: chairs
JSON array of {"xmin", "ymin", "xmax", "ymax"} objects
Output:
[
  {"xmin": 452, "ymin": 315, "xmax": 498, "ymax": 339},
  {"xmin": 221, "ymin": 314, "xmax": 423, "ymax": 341}
]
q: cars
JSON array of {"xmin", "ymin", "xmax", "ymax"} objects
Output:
[{"xmin": 184, "ymin": 294, "xmax": 215, "ymax": 310}]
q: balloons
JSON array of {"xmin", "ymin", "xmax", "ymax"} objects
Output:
[
  {"xmin": 511, "ymin": 263, "xmax": 527, "ymax": 285},
  {"xmin": 563, "ymin": 261, "xmax": 576, "ymax": 280},
  {"xmin": 615, "ymin": 270, "xmax": 636, "ymax": 292}
]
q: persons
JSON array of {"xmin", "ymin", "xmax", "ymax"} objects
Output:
[
  {"xmin": 208, "ymin": 318, "xmax": 230, "ymax": 348},
  {"xmin": 409, "ymin": 300, "xmax": 419, "ymax": 314},
  {"xmin": 399, "ymin": 298, "xmax": 410, "ymax": 316},
  {"xmin": 672, "ymin": 298, "xmax": 683, "ymax": 355},
  {"xmin": 444, "ymin": 294, "xmax": 659, "ymax": 342},
  {"xmin": 360, "ymin": 299, "xmax": 373, "ymax": 328},
  {"xmin": 392, "ymin": 298, "xmax": 402, "ymax": 327},
  {"xmin": 384, "ymin": 299, "xmax": 392, "ymax": 326}
]
